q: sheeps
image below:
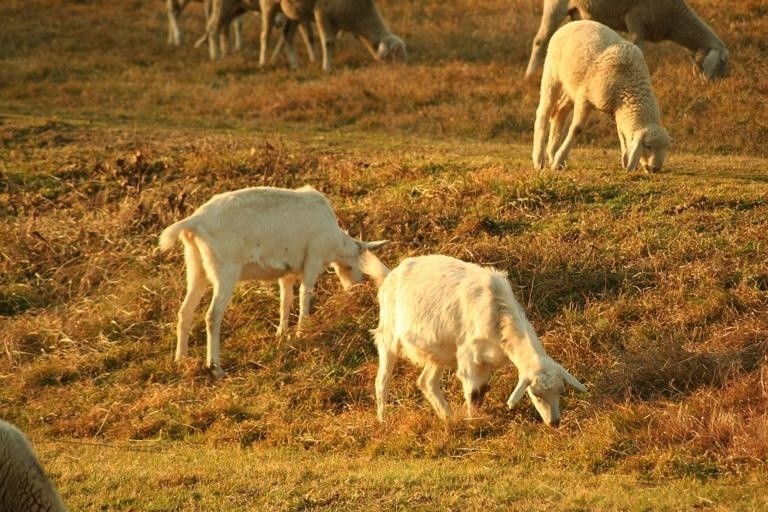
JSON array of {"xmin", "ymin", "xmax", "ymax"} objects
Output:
[
  {"xmin": 160, "ymin": 0, "xmax": 408, "ymax": 75},
  {"xmin": 518, "ymin": 3, "xmax": 733, "ymax": 174},
  {"xmin": 158, "ymin": 181, "xmax": 391, "ymax": 384},
  {"xmin": 355, "ymin": 248, "xmax": 589, "ymax": 429},
  {"xmin": 0, "ymin": 416, "xmax": 68, "ymax": 512}
]
